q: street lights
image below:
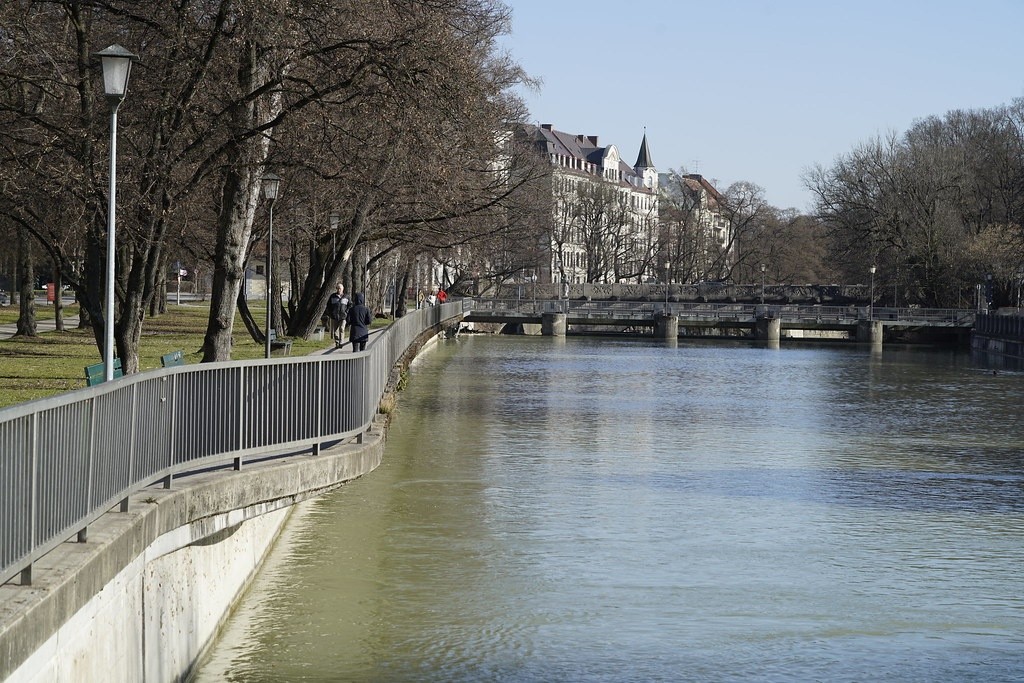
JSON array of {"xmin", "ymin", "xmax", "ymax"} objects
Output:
[
  {"xmin": 94, "ymin": 41, "xmax": 140, "ymax": 382},
  {"xmin": 329, "ymin": 209, "xmax": 339, "ymax": 339},
  {"xmin": 871, "ymin": 264, "xmax": 876, "ymax": 320},
  {"xmin": 976, "ymin": 283, "xmax": 981, "ymax": 314},
  {"xmin": 664, "ymin": 259, "xmax": 670, "ymax": 315},
  {"xmin": 760, "ymin": 263, "xmax": 765, "ymax": 304},
  {"xmin": 260, "ymin": 172, "xmax": 280, "ymax": 358}
]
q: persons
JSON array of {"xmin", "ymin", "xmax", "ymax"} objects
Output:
[
  {"xmin": 418, "ymin": 289, "xmax": 425, "ymax": 307},
  {"xmin": 351, "ymin": 292, "xmax": 372, "ymax": 351},
  {"xmin": 438, "ymin": 287, "xmax": 447, "ymax": 304},
  {"xmin": 327, "ymin": 283, "xmax": 348, "ymax": 348},
  {"xmin": 427, "ymin": 290, "xmax": 437, "ymax": 306}
]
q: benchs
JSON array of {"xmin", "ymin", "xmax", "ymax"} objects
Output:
[
  {"xmin": 84, "ymin": 358, "xmax": 124, "ymax": 387},
  {"xmin": 161, "ymin": 350, "xmax": 184, "ymax": 368},
  {"xmin": 270, "ymin": 329, "xmax": 292, "ymax": 356}
]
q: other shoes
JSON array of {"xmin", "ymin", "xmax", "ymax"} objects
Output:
[
  {"xmin": 335, "ymin": 346, "xmax": 338, "ymax": 349},
  {"xmin": 339, "ymin": 344, "xmax": 342, "ymax": 348}
]
